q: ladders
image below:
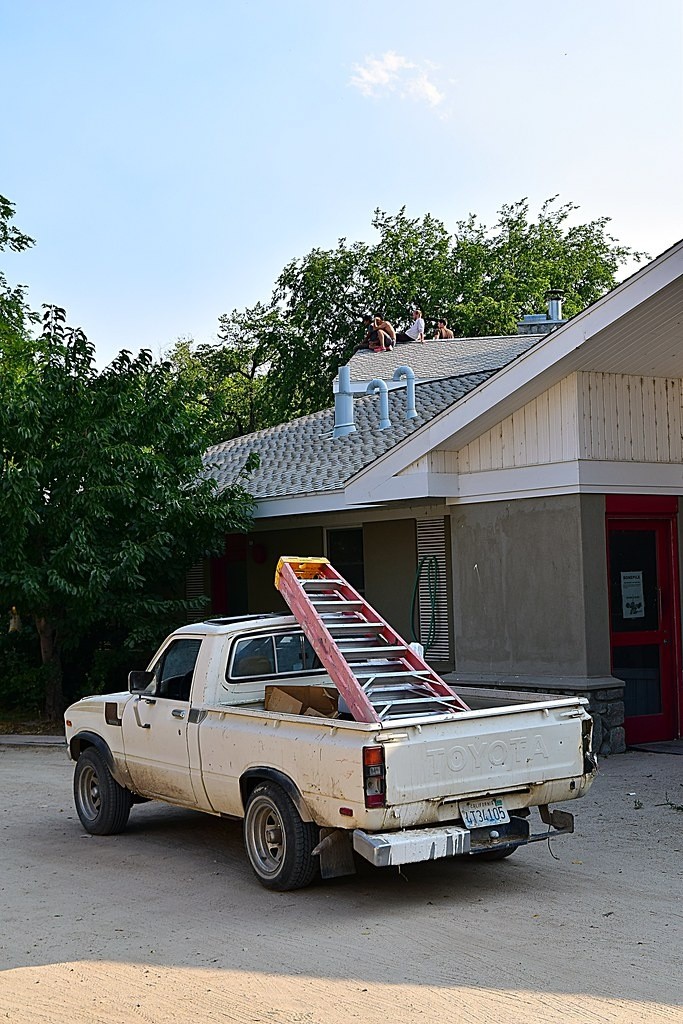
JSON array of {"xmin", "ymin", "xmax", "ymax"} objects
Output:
[{"xmin": 272, "ymin": 546, "xmax": 474, "ymax": 731}]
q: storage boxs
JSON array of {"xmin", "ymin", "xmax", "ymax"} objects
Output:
[{"xmin": 264, "ymin": 685, "xmax": 339, "ymax": 722}]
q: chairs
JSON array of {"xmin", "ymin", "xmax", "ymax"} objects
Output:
[{"xmin": 235, "ymin": 656, "xmax": 272, "ymax": 676}]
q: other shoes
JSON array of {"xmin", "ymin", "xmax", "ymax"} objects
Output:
[
  {"xmin": 374, "ymin": 347, "xmax": 386, "ymax": 352},
  {"xmin": 389, "ymin": 345, "xmax": 392, "ymax": 351}
]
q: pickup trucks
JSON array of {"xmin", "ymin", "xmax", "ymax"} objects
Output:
[{"xmin": 63, "ymin": 613, "xmax": 598, "ymax": 892}]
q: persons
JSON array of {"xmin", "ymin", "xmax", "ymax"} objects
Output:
[
  {"xmin": 396, "ymin": 310, "xmax": 427, "ymax": 344},
  {"xmin": 435, "ymin": 318, "xmax": 453, "ymax": 339},
  {"xmin": 353, "ymin": 313, "xmax": 396, "ymax": 352}
]
